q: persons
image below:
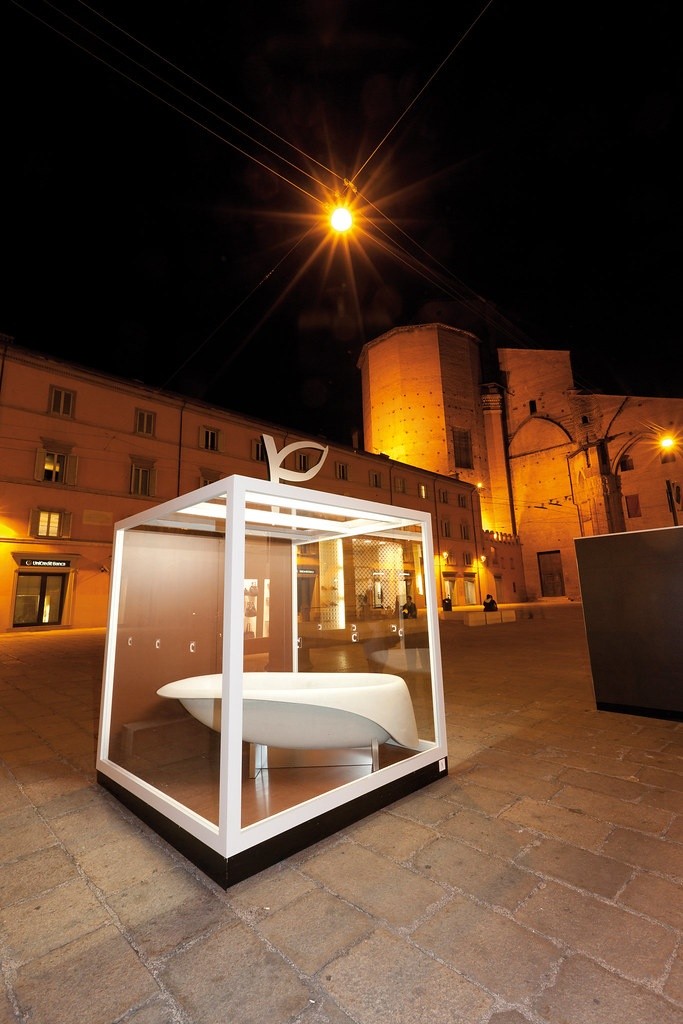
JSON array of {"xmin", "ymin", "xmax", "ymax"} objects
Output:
[
  {"xmin": 402, "ymin": 595, "xmax": 417, "ymax": 620},
  {"xmin": 482, "ymin": 594, "xmax": 498, "ymax": 612}
]
